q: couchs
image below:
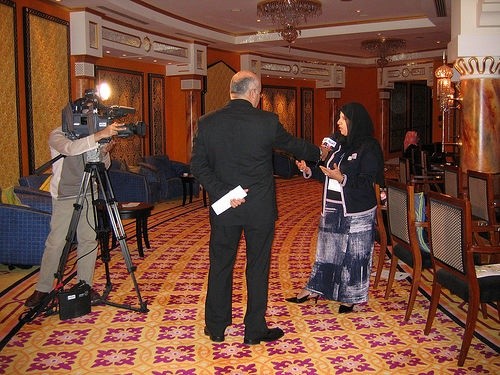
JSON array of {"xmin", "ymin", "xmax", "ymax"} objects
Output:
[{"xmin": 0, "ymin": 155, "xmax": 190, "ymax": 266}]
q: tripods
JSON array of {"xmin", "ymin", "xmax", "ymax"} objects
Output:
[{"xmin": 46, "ymin": 162, "xmax": 148, "ymax": 313}]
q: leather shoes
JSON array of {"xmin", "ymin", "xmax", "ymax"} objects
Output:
[
  {"xmin": 204, "ymin": 326, "xmax": 225, "ymax": 342},
  {"xmin": 243, "ymin": 327, "xmax": 285, "ymax": 345}
]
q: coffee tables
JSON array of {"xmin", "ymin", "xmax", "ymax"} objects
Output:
[
  {"xmin": 182, "ymin": 173, "xmax": 207, "ymax": 207},
  {"xmin": 102, "ymin": 202, "xmax": 154, "ymax": 257}
]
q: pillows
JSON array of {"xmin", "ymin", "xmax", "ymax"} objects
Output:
[
  {"xmin": 39, "ymin": 175, "xmax": 52, "ymax": 191},
  {"xmin": 2, "ymin": 186, "xmax": 21, "ymax": 206},
  {"xmin": 414, "ymin": 190, "xmax": 431, "ymax": 254},
  {"xmin": 128, "ymin": 165, "xmax": 141, "ymax": 173}
]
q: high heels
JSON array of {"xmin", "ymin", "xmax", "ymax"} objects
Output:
[
  {"xmin": 338, "ymin": 303, "xmax": 355, "ymax": 313},
  {"xmin": 285, "ymin": 292, "xmax": 318, "ymax": 304}
]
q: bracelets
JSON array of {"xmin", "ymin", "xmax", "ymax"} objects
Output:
[
  {"xmin": 319, "ymin": 145, "xmax": 323, "ymax": 155},
  {"xmin": 338, "ymin": 175, "xmax": 345, "ymax": 185}
]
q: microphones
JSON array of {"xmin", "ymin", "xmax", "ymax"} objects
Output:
[{"xmin": 314, "ymin": 130, "xmax": 341, "ymax": 168}]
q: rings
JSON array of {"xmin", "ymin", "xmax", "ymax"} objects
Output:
[{"xmin": 325, "ymin": 173, "xmax": 327, "ymax": 176}]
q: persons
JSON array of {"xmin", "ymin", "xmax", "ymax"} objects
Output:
[
  {"xmin": 24, "ymin": 99, "xmax": 126, "ymax": 307},
  {"xmin": 403, "ymin": 124, "xmax": 420, "ymax": 153},
  {"xmin": 284, "ymin": 102, "xmax": 383, "ymax": 313},
  {"xmin": 189, "ymin": 69, "xmax": 327, "ymax": 345}
]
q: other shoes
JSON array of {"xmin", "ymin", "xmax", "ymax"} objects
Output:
[
  {"xmin": 90, "ymin": 288, "xmax": 101, "ymax": 300},
  {"xmin": 25, "ymin": 290, "xmax": 49, "ymax": 307}
]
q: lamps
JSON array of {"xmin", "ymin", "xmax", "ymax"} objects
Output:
[
  {"xmin": 256, "ymin": 1, "xmax": 322, "ymax": 53},
  {"xmin": 361, "ymin": 38, "xmax": 407, "ymax": 71}
]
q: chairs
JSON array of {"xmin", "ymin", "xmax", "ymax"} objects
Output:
[{"xmin": 373, "ymin": 138, "xmax": 500, "ymax": 367}]
